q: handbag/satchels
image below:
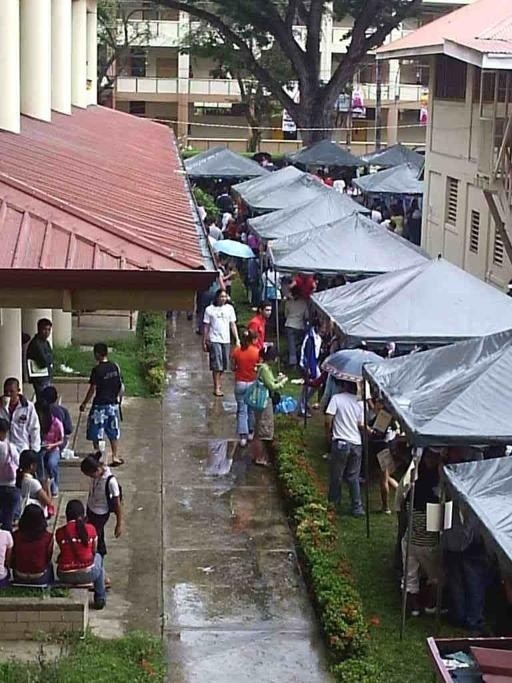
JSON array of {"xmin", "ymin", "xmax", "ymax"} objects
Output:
[
  {"xmin": 18, "ymin": 479, "xmax": 48, "ymax": 520},
  {"xmin": 243, "ymin": 380, "xmax": 270, "ymax": 411}
]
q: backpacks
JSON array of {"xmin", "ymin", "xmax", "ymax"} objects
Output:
[{"xmin": 105, "ymin": 475, "xmax": 122, "ymax": 513}]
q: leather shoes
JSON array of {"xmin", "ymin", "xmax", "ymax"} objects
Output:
[
  {"xmin": 111, "ymin": 458, "xmax": 124, "ymax": 467},
  {"xmin": 240, "ymin": 438, "xmax": 247, "ymax": 448},
  {"xmin": 298, "ymin": 410, "xmax": 312, "ymax": 417},
  {"xmin": 248, "ymin": 432, "xmax": 255, "ymax": 442}
]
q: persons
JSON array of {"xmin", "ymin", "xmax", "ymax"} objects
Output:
[
  {"xmin": 80, "ymin": 343, "xmax": 125, "ymax": 467},
  {"xmin": 0, "ymin": 377, "xmax": 73, "ymax": 586},
  {"xmin": 80, "ymin": 451, "xmax": 124, "ymax": 591},
  {"xmin": 26, "ymin": 318, "xmax": 55, "ymax": 403},
  {"xmin": 55, "ymin": 500, "xmax": 107, "ymax": 609},
  {"xmin": 187, "ymin": 162, "xmax": 491, "ymax": 630}
]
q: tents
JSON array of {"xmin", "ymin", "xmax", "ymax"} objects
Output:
[{"xmin": 184, "ymin": 139, "xmax": 512, "ymax": 572}]
